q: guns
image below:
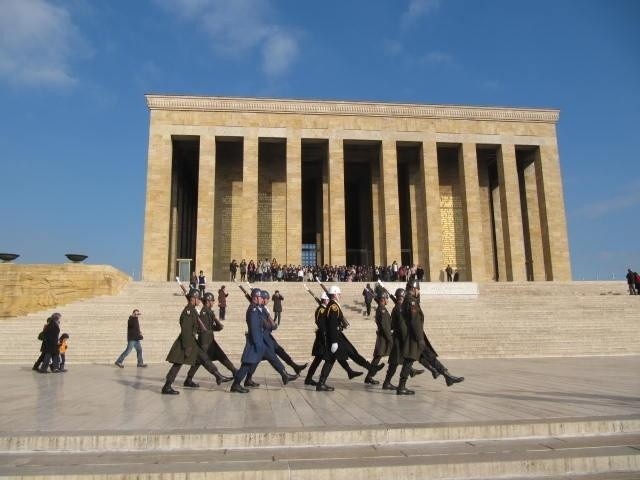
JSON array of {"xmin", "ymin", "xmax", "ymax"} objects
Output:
[
  {"xmin": 176, "ymin": 277, "xmax": 223, "ymax": 332},
  {"xmin": 237, "ymin": 282, "xmax": 278, "ymax": 329},
  {"xmin": 364, "ymin": 281, "xmax": 398, "ymax": 305},
  {"xmin": 305, "ymin": 275, "xmax": 350, "ymax": 330}
]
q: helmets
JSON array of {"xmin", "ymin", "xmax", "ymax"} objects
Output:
[
  {"xmin": 395, "ymin": 278, "xmax": 419, "ymax": 297},
  {"xmin": 377, "ymin": 290, "xmax": 387, "ymax": 298},
  {"xmin": 251, "ymin": 288, "xmax": 269, "ymax": 299},
  {"xmin": 320, "ymin": 286, "xmax": 340, "ymax": 300},
  {"xmin": 189, "ymin": 289, "xmax": 214, "ymax": 301}
]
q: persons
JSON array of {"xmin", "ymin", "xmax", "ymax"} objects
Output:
[
  {"xmin": 191, "ymin": 270, "xmax": 207, "ymax": 297},
  {"xmin": 115, "ymin": 309, "xmax": 148, "ymax": 368},
  {"xmin": 58, "ymin": 334, "xmax": 69, "ymax": 373},
  {"xmin": 304, "ymin": 285, "xmax": 385, "ymax": 391},
  {"xmin": 161, "ymin": 289, "xmax": 238, "ymax": 396},
  {"xmin": 41, "ymin": 312, "xmax": 62, "ymax": 373},
  {"xmin": 218, "ymin": 285, "xmax": 229, "ymax": 320},
  {"xmin": 626, "ymin": 268, "xmax": 636, "ymax": 295},
  {"xmin": 32, "ymin": 317, "xmax": 52, "ymax": 370},
  {"xmin": 633, "ymin": 272, "xmax": 640, "ymax": 296},
  {"xmin": 362, "ymin": 281, "xmax": 465, "ymax": 397},
  {"xmin": 445, "ymin": 264, "xmax": 459, "ymax": 282},
  {"xmin": 230, "ymin": 257, "xmax": 424, "ymax": 282},
  {"xmin": 230, "ymin": 287, "xmax": 308, "ymax": 394}
]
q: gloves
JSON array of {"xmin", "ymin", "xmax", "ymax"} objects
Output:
[{"xmin": 331, "ymin": 343, "xmax": 338, "ymax": 354}]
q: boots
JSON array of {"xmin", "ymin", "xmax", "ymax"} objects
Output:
[
  {"xmin": 428, "ymin": 365, "xmax": 464, "ymax": 386},
  {"xmin": 213, "ymin": 365, "xmax": 259, "ymax": 393},
  {"xmin": 184, "ymin": 376, "xmax": 199, "ymax": 387},
  {"xmin": 162, "ymin": 380, "xmax": 179, "ymax": 394},
  {"xmin": 346, "ymin": 367, "xmax": 363, "ymax": 379},
  {"xmin": 281, "ymin": 363, "xmax": 334, "ymax": 391},
  {"xmin": 365, "ymin": 361, "xmax": 424, "ymax": 395}
]
